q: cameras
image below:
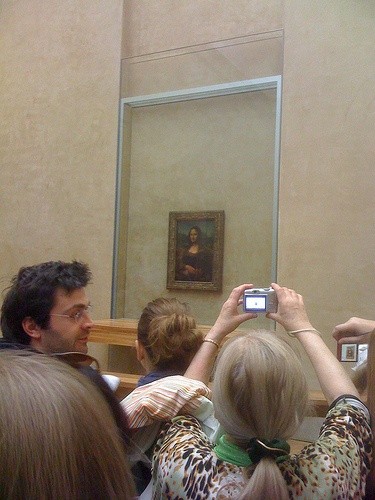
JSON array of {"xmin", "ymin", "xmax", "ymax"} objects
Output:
[
  {"xmin": 243, "ymin": 288, "xmax": 279, "ymax": 313},
  {"xmin": 337, "ymin": 341, "xmax": 368, "ymax": 364}
]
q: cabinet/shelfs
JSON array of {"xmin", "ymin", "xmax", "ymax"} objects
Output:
[{"xmin": 87, "ymin": 317, "xmax": 368, "ymax": 418}]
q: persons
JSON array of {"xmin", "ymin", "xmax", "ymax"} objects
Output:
[
  {"xmin": 152, "ymin": 283, "xmax": 371, "ymax": 500},
  {"xmin": 332, "ymin": 316, "xmax": 375, "ymax": 422},
  {"xmin": 0, "ymin": 259, "xmax": 92, "ymax": 355},
  {"xmin": 0, "ymin": 350, "xmax": 136, "ymax": 500},
  {"xmin": 135, "ymin": 297, "xmax": 202, "ymax": 386}
]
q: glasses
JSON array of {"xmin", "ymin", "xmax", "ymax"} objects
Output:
[
  {"xmin": 48, "ymin": 304, "xmax": 96, "ymax": 321},
  {"xmin": 49, "ymin": 351, "xmax": 100, "ymax": 375}
]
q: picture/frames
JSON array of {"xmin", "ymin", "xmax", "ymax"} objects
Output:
[{"xmin": 166, "ymin": 210, "xmax": 225, "ymax": 292}]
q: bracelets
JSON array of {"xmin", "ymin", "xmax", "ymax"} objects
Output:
[
  {"xmin": 288, "ymin": 329, "xmax": 322, "ymax": 336},
  {"xmin": 202, "ymin": 339, "xmax": 219, "ymax": 346}
]
